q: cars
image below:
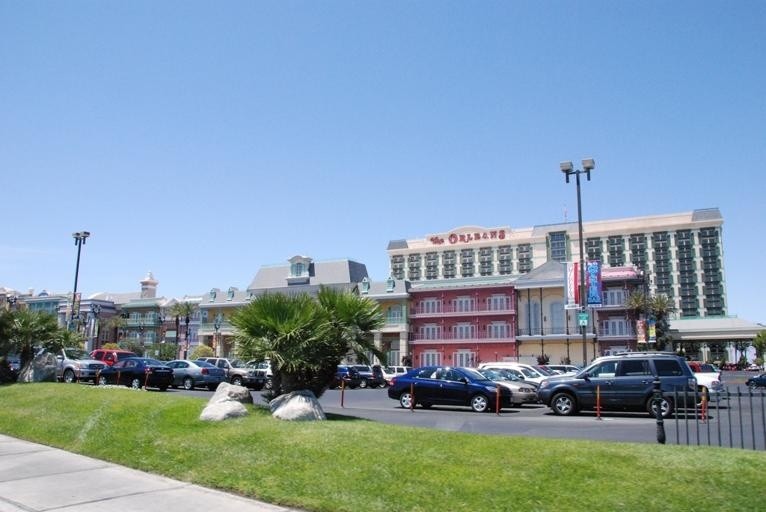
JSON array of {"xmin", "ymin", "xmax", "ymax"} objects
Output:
[
  {"xmin": 88, "ymin": 348, "xmax": 137, "ymax": 367},
  {"xmin": 165, "ymin": 359, "xmax": 226, "ymax": 390},
  {"xmin": 3, "ymin": 356, "xmax": 24, "ymax": 382},
  {"xmin": 37, "ymin": 348, "xmax": 109, "ymax": 384},
  {"xmin": 477, "ymin": 354, "xmax": 721, "ymax": 407},
  {"xmin": 98, "ymin": 356, "xmax": 176, "ymax": 390},
  {"xmin": 388, "ymin": 365, "xmax": 513, "ymax": 414},
  {"xmin": 745, "ymin": 372, "xmax": 766, "ymax": 389}
]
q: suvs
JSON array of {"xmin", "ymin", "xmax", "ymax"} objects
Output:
[
  {"xmin": 195, "ymin": 357, "xmax": 274, "ymax": 390},
  {"xmin": 537, "ymin": 352, "xmax": 701, "ymax": 417},
  {"xmin": 336, "ymin": 364, "xmax": 415, "ymax": 389}
]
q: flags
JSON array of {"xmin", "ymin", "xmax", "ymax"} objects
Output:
[
  {"xmin": 636, "ymin": 318, "xmax": 648, "ymax": 344},
  {"xmin": 647, "ymin": 319, "xmax": 657, "ymax": 343},
  {"xmin": 584, "ymin": 259, "xmax": 604, "ymax": 308},
  {"xmin": 562, "ymin": 262, "xmax": 581, "ymax": 310}
]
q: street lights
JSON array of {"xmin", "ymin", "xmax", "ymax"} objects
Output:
[
  {"xmin": 560, "ymin": 158, "xmax": 595, "ymax": 368},
  {"xmin": 6, "ymin": 294, "xmax": 19, "ymax": 309},
  {"xmin": 635, "ymin": 268, "xmax": 653, "ymax": 318},
  {"xmin": 69, "ymin": 231, "xmax": 91, "ymax": 324}
]
